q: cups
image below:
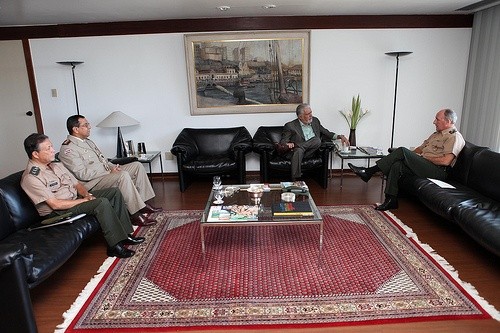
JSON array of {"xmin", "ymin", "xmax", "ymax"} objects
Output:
[
  {"xmin": 343, "ymin": 142, "xmax": 349, "ymax": 152},
  {"xmin": 212, "ymin": 176, "xmax": 222, "ymax": 188},
  {"xmin": 138, "ymin": 142, "xmax": 146, "ymax": 154}
]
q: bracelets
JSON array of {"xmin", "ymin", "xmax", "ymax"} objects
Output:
[{"xmin": 84, "ymin": 193, "xmax": 93, "ymax": 196}]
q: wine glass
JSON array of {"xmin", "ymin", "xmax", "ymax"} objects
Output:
[{"xmin": 213, "ymin": 188, "xmax": 225, "ymax": 204}]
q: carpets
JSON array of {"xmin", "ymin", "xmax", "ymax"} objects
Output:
[{"xmin": 55, "ymin": 202, "xmax": 500, "ymax": 333}]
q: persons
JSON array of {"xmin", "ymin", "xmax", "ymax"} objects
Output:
[
  {"xmin": 58, "ymin": 115, "xmax": 163, "ymax": 226},
  {"xmin": 21, "ymin": 133, "xmax": 145, "ymax": 257},
  {"xmin": 347, "ymin": 108, "xmax": 465, "ymax": 211},
  {"xmin": 275, "ymin": 103, "xmax": 350, "ymax": 181}
]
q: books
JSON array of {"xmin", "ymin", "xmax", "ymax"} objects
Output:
[
  {"xmin": 280, "ymin": 181, "xmax": 309, "ymax": 195},
  {"xmin": 272, "ymin": 201, "xmax": 313, "ymax": 217},
  {"xmin": 357, "ymin": 146, "xmax": 383, "ymax": 155}
]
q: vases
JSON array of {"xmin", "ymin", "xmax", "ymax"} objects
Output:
[{"xmin": 349, "ymin": 129, "xmax": 356, "ymax": 147}]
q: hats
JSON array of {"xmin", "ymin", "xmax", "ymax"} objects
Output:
[{"xmin": 41, "ymin": 211, "xmax": 73, "ymax": 225}]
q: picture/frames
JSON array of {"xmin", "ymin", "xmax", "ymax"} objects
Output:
[{"xmin": 184, "ymin": 30, "xmax": 312, "ymax": 116}]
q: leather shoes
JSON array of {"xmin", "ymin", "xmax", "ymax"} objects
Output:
[
  {"xmin": 348, "ymin": 162, "xmax": 372, "ymax": 183},
  {"xmin": 131, "ymin": 214, "xmax": 157, "ymax": 226},
  {"xmin": 140, "ymin": 203, "xmax": 163, "ymax": 213},
  {"xmin": 107, "ymin": 243, "xmax": 135, "ymax": 258},
  {"xmin": 275, "ymin": 144, "xmax": 289, "ymax": 155},
  {"xmin": 122, "ymin": 233, "xmax": 145, "ymax": 244},
  {"xmin": 374, "ymin": 198, "xmax": 399, "ymax": 211}
]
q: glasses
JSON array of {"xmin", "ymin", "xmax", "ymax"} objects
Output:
[{"xmin": 76, "ymin": 123, "xmax": 90, "ymax": 127}]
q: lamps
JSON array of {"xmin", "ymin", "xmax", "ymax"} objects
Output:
[
  {"xmin": 55, "ymin": 61, "xmax": 85, "ymax": 115},
  {"xmin": 95, "ymin": 110, "xmax": 139, "ymax": 157},
  {"xmin": 380, "ymin": 51, "xmax": 413, "ymax": 180}
]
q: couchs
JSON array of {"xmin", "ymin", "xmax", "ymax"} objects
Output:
[
  {"xmin": 253, "ymin": 126, "xmax": 335, "ymax": 189},
  {"xmin": 171, "ymin": 126, "xmax": 253, "ymax": 192},
  {"xmin": 398, "ymin": 139, "xmax": 500, "ymax": 254},
  {"xmin": 0, "ymin": 153, "xmax": 139, "ymax": 333}
]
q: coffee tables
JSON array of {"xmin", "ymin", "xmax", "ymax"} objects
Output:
[
  {"xmin": 200, "ymin": 181, "xmax": 324, "ymax": 253},
  {"xmin": 330, "ymin": 145, "xmax": 390, "ymax": 188},
  {"xmin": 107, "ymin": 151, "xmax": 166, "ymax": 187}
]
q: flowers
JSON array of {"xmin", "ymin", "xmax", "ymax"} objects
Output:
[{"xmin": 339, "ymin": 95, "xmax": 369, "ymax": 128}]
